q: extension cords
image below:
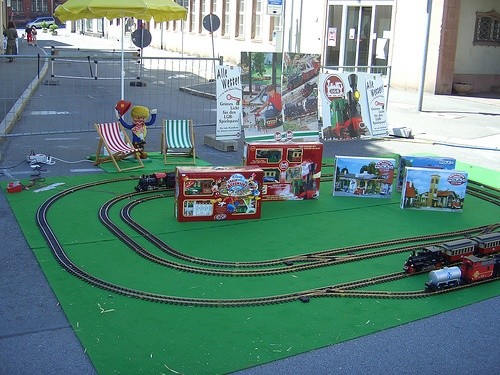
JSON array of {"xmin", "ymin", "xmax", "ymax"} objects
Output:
[{"xmin": 27, "ymin": 154, "xmax": 46, "ymax": 162}]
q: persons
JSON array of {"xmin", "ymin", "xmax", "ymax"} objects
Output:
[
  {"xmin": 25, "ymin": 24, "xmax": 37, "ymax": 47},
  {"xmin": 256, "ymin": 84, "xmax": 282, "ymax": 118},
  {"xmin": 3, "ymin": 22, "xmax": 18, "ymax": 62}
]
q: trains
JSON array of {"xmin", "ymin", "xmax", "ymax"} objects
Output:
[
  {"xmin": 134, "ymin": 171, "xmax": 176, "ymax": 190},
  {"xmin": 402, "ymin": 233, "xmax": 500, "ymax": 273},
  {"xmin": 424, "ymin": 253, "xmax": 500, "ymax": 294},
  {"xmin": 322, "ymin": 72, "xmax": 370, "ymax": 140}
]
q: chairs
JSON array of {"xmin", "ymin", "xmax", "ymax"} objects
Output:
[
  {"xmin": 161, "ymin": 120, "xmax": 196, "ymax": 165},
  {"xmin": 94, "ymin": 122, "xmax": 145, "ymax": 172}
]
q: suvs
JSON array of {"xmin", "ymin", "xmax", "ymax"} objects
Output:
[{"xmin": 25, "ymin": 16, "xmax": 56, "ymax": 30}]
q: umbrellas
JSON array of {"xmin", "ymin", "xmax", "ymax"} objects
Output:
[{"xmin": 53, "ymin": 0, "xmax": 187, "ymax": 120}]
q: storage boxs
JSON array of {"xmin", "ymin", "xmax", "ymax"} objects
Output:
[
  {"xmin": 397, "ymin": 156, "xmax": 456, "ymax": 191},
  {"xmin": 243, "ymin": 141, "xmax": 323, "ymax": 200},
  {"xmin": 175, "ymin": 165, "xmax": 265, "ymax": 221},
  {"xmin": 399, "ymin": 167, "xmax": 468, "ymax": 212},
  {"xmin": 332, "ymin": 154, "xmax": 396, "ymax": 199}
]
